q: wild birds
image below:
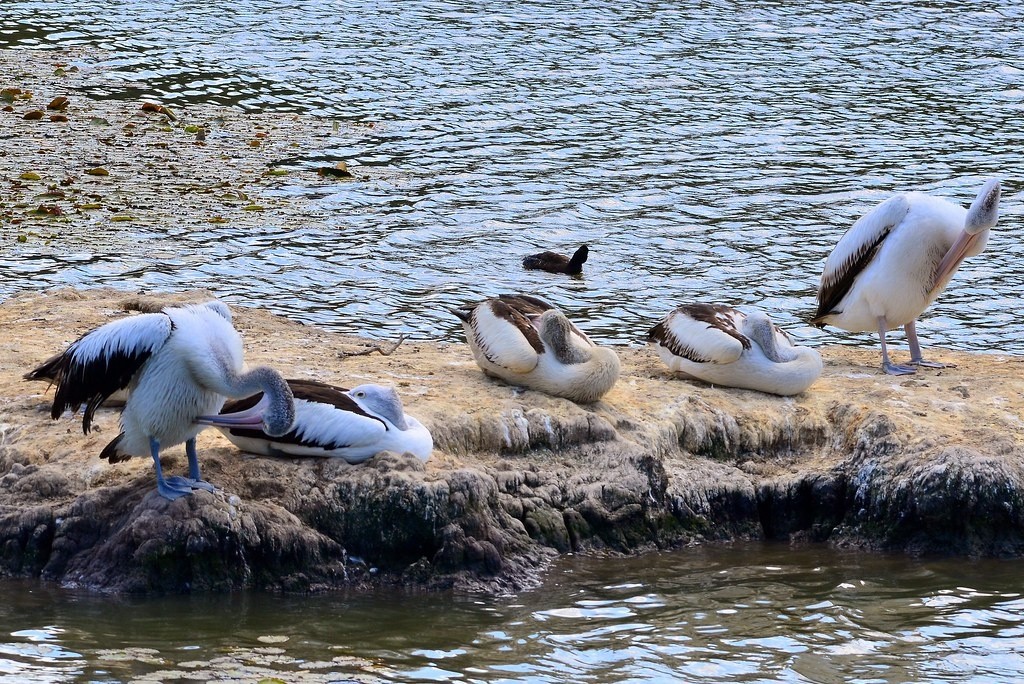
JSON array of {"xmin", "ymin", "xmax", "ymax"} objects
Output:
[
  {"xmin": 439, "ymin": 293, "xmax": 621, "ymax": 404},
  {"xmin": 637, "ymin": 305, "xmax": 823, "ymax": 396},
  {"xmin": 802, "ymin": 177, "xmax": 1001, "ymax": 376},
  {"xmin": 522, "ymin": 245, "xmax": 589, "ymax": 274},
  {"xmin": 213, "ymin": 379, "xmax": 433, "ymax": 463},
  {"xmin": 21, "ymin": 300, "xmax": 295, "ymax": 502}
]
